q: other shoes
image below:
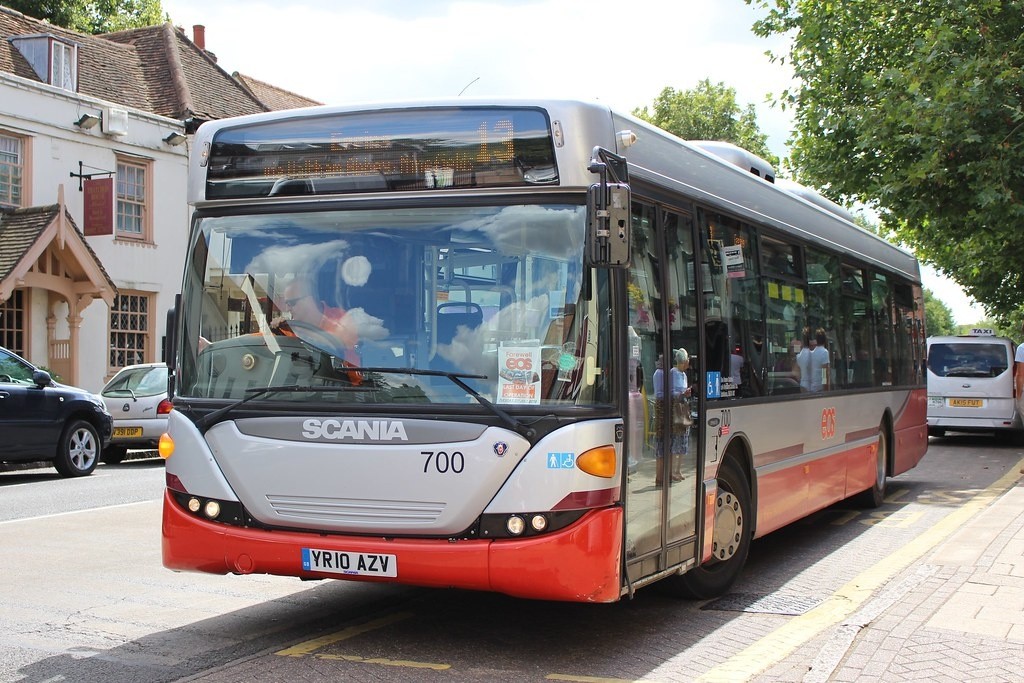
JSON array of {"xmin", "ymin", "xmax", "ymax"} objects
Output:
[
  {"xmin": 672, "ymin": 472, "xmax": 685, "ymax": 481},
  {"xmin": 655, "ymin": 477, "xmax": 664, "ymax": 486}
]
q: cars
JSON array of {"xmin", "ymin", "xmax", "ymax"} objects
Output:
[
  {"xmin": 0, "ymin": 344, "xmax": 114, "ymax": 477},
  {"xmin": 100, "ymin": 362, "xmax": 177, "ymax": 466}
]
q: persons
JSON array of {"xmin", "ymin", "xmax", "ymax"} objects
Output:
[
  {"xmin": 813, "ymin": 326, "xmax": 832, "ymax": 392},
  {"xmin": 653, "ymin": 355, "xmax": 664, "ymax": 486},
  {"xmin": 1015, "ymin": 341, "xmax": 1024, "ymax": 474},
  {"xmin": 797, "ymin": 327, "xmax": 812, "ymax": 393},
  {"xmin": 195, "ymin": 274, "xmax": 365, "ymax": 387},
  {"xmin": 670, "ymin": 347, "xmax": 695, "ymax": 482}
]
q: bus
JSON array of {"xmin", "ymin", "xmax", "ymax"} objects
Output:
[{"xmin": 156, "ymin": 99, "xmax": 930, "ymax": 602}]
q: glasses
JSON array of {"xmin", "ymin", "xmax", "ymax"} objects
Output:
[{"xmin": 286, "ymin": 294, "xmax": 307, "ymax": 306}]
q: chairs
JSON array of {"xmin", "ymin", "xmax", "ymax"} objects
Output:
[{"xmin": 426, "ymin": 300, "xmax": 484, "ymax": 392}]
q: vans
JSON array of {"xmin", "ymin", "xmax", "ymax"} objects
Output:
[{"xmin": 925, "ymin": 329, "xmax": 1018, "ymax": 437}]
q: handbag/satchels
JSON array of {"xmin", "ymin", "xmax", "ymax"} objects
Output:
[
  {"xmin": 655, "ymin": 396, "xmax": 665, "ymax": 437},
  {"xmin": 672, "ymin": 393, "xmax": 693, "ymax": 435}
]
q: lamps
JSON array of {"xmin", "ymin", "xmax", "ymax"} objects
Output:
[
  {"xmin": 74, "ymin": 112, "xmax": 103, "ymax": 130},
  {"xmin": 162, "ymin": 132, "xmax": 186, "ymax": 146}
]
